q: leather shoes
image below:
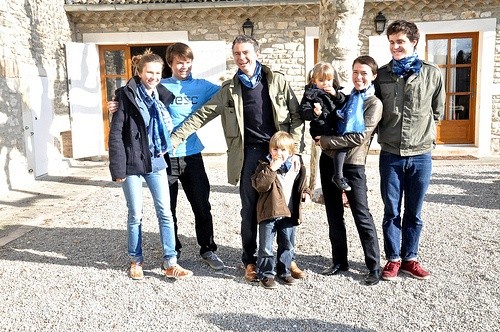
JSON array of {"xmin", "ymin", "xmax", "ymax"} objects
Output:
[
  {"xmin": 322, "ymin": 263, "xmax": 348, "ymax": 275},
  {"xmin": 365, "ymin": 266, "xmax": 382, "ymax": 286}
]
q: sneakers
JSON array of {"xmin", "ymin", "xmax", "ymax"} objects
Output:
[
  {"xmin": 401, "ymin": 260, "xmax": 430, "ymax": 280},
  {"xmin": 380, "ymin": 259, "xmax": 402, "ymax": 281},
  {"xmin": 332, "ymin": 175, "xmax": 351, "ymax": 192},
  {"xmin": 203, "ymin": 251, "xmax": 225, "ymax": 270},
  {"xmin": 166, "ymin": 266, "xmax": 193, "ymax": 279},
  {"xmin": 131, "ymin": 262, "xmax": 143, "ymax": 280}
]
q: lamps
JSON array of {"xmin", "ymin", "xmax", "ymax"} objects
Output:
[
  {"xmin": 243, "ymin": 18, "xmax": 254, "ymax": 37},
  {"xmin": 374, "ymin": 11, "xmax": 387, "ymax": 35}
]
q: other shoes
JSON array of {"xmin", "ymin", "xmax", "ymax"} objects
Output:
[
  {"xmin": 245, "ymin": 264, "xmax": 260, "ymax": 282},
  {"xmin": 290, "ymin": 261, "xmax": 304, "ymax": 279},
  {"xmin": 281, "ymin": 275, "xmax": 296, "ymax": 284},
  {"xmin": 261, "ymin": 278, "xmax": 277, "ymax": 289}
]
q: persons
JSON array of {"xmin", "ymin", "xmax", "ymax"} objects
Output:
[
  {"xmin": 251, "ymin": 131, "xmax": 312, "ymax": 290},
  {"xmin": 301, "ymin": 62, "xmax": 353, "ymax": 192},
  {"xmin": 315, "ymin": 20, "xmax": 446, "ymax": 286},
  {"xmin": 107, "ymin": 41, "xmax": 223, "ymax": 280},
  {"xmin": 169, "ymin": 36, "xmax": 307, "ymax": 283}
]
